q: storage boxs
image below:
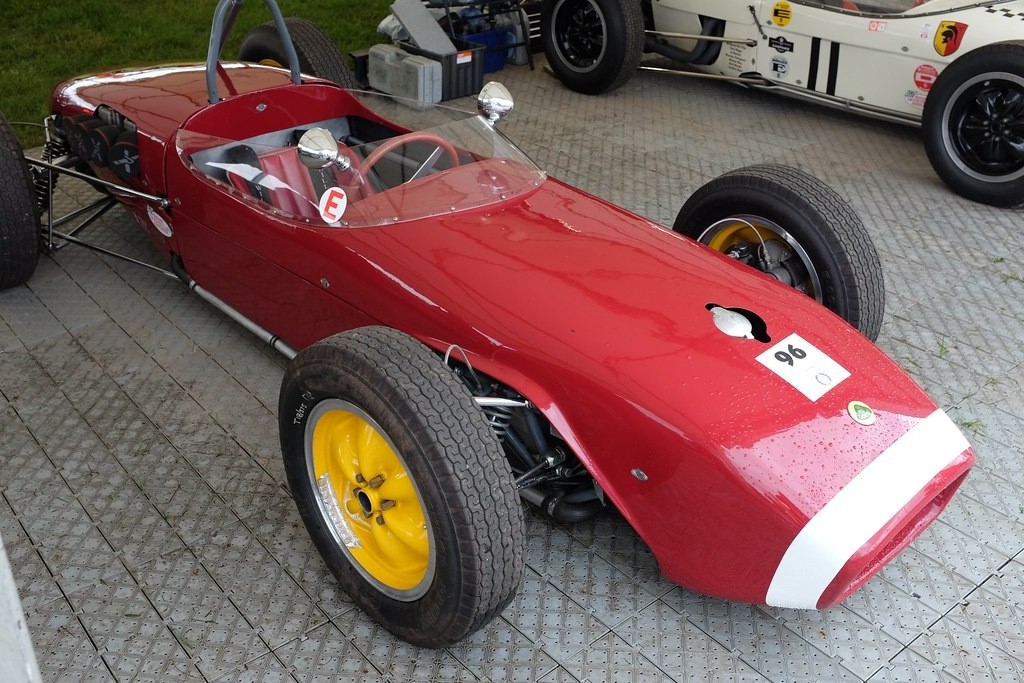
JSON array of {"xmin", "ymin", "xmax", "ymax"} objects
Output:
[
  {"xmin": 444, "ymin": 25, "xmax": 512, "ymax": 74},
  {"xmin": 368, "ymin": 44, "xmax": 443, "ymax": 111},
  {"xmin": 396, "ymin": 38, "xmax": 488, "ymax": 102}
]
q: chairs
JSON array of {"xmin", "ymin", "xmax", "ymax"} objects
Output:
[{"xmin": 227, "ymin": 139, "xmax": 379, "ymax": 219}]
